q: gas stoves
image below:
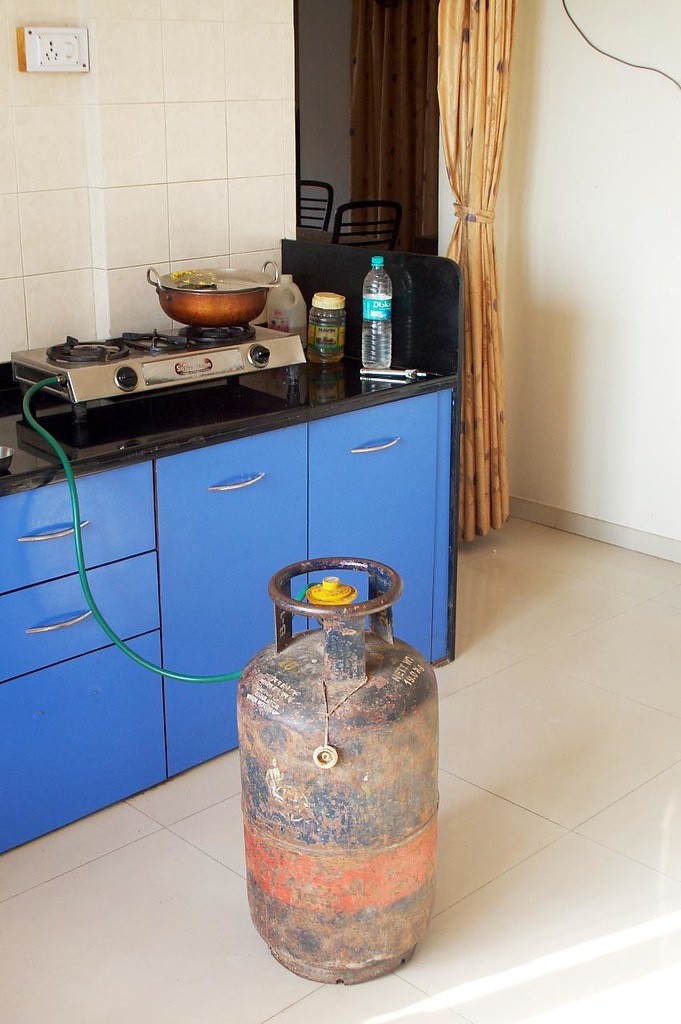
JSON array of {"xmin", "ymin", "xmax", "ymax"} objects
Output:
[{"xmin": 11, "ymin": 321, "xmax": 308, "ymax": 404}]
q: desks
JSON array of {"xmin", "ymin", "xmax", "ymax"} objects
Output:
[{"xmin": 296, "ymin": 227, "xmax": 419, "ymax": 254}]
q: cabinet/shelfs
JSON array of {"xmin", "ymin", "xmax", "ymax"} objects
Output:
[{"xmin": 0, "ymin": 384, "xmax": 459, "ymax": 851}]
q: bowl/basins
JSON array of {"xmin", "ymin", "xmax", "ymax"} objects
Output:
[{"xmin": 0, "ymin": 446, "xmax": 15, "ymax": 472}]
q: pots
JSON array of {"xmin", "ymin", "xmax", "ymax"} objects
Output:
[{"xmin": 146, "ymin": 261, "xmax": 278, "ymax": 328}]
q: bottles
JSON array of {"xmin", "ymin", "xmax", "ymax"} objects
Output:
[
  {"xmin": 359, "ymin": 254, "xmax": 392, "ymax": 370},
  {"xmin": 266, "ymin": 274, "xmax": 346, "ymax": 363}
]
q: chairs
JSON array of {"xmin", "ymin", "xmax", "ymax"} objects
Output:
[
  {"xmin": 298, "ymin": 180, "xmax": 334, "ymax": 231},
  {"xmin": 331, "ymin": 200, "xmax": 403, "ymax": 250}
]
